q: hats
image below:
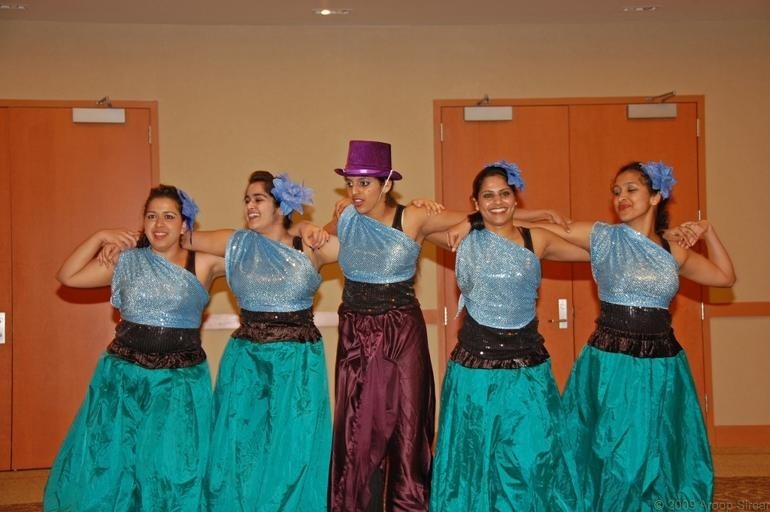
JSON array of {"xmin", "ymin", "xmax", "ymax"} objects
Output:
[{"xmin": 334, "ymin": 140, "xmax": 402, "ymax": 180}]
